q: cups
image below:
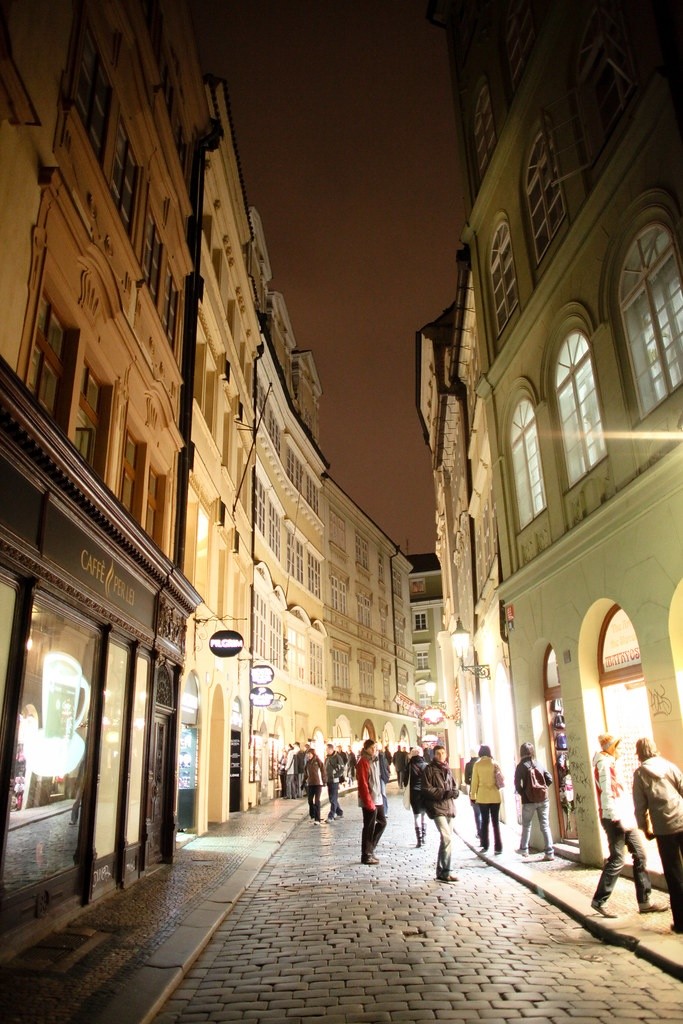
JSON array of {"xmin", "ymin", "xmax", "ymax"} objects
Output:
[{"xmin": 42, "ymin": 651, "xmax": 90, "ymax": 747}]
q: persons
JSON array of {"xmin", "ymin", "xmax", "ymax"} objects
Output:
[
  {"xmin": 629, "ymin": 737, "xmax": 683, "ymax": 937},
  {"xmin": 421, "ymin": 745, "xmax": 459, "ymax": 882},
  {"xmin": 403, "ymin": 746, "xmax": 428, "ymax": 849},
  {"xmin": 334, "ymin": 744, "xmax": 432, "ymax": 789},
  {"xmin": 355, "ymin": 739, "xmax": 387, "ymax": 865},
  {"xmin": 514, "ymin": 742, "xmax": 555, "ymax": 861},
  {"xmin": 323, "ymin": 744, "xmax": 344, "ymax": 823},
  {"xmin": 301, "ymin": 748, "xmax": 328, "ymax": 826},
  {"xmin": 465, "ymin": 744, "xmax": 504, "ymax": 855},
  {"xmin": 276, "ymin": 741, "xmax": 312, "ymax": 799},
  {"xmin": 591, "ymin": 732, "xmax": 670, "ymax": 918}
]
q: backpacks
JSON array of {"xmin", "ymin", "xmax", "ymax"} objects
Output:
[{"xmin": 522, "ymin": 760, "xmax": 548, "ymax": 803}]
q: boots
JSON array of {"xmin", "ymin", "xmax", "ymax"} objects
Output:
[
  {"xmin": 422, "ymin": 822, "xmax": 427, "ymax": 844},
  {"xmin": 415, "ymin": 827, "xmax": 422, "ymax": 847}
]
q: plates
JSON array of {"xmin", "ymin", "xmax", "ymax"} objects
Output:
[{"xmin": 23, "ymin": 729, "xmax": 85, "ymax": 778}]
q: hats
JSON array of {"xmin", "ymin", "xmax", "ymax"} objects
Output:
[
  {"xmin": 552, "ymin": 698, "xmax": 574, "ymax": 830},
  {"xmin": 308, "ymin": 749, "xmax": 315, "ymax": 755},
  {"xmin": 599, "ymin": 733, "xmax": 621, "ymax": 757},
  {"xmin": 412, "ymin": 746, "xmax": 423, "ymax": 757}
]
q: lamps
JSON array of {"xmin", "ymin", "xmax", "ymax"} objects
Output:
[
  {"xmin": 450, "ymin": 616, "xmax": 492, "ymax": 681},
  {"xmin": 423, "ymin": 673, "xmax": 447, "ymax": 711}
]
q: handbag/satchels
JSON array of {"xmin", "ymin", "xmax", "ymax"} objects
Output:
[
  {"xmin": 339, "ymin": 774, "xmax": 345, "ymax": 782},
  {"xmin": 495, "ymin": 764, "xmax": 504, "ymax": 788},
  {"xmin": 403, "ymin": 781, "xmax": 411, "ymax": 810}
]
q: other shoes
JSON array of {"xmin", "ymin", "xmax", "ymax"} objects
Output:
[
  {"xmin": 314, "ymin": 820, "xmax": 320, "ymax": 825},
  {"xmin": 361, "ymin": 856, "xmax": 378, "ymax": 864},
  {"xmin": 641, "ymin": 902, "xmax": 668, "ymax": 912},
  {"xmin": 334, "ymin": 811, "xmax": 343, "ymax": 820},
  {"xmin": 671, "ymin": 924, "xmax": 683, "ymax": 934},
  {"xmin": 515, "ymin": 848, "xmax": 528, "ymax": 856},
  {"xmin": 438, "ymin": 875, "xmax": 457, "ymax": 881},
  {"xmin": 591, "ymin": 900, "xmax": 617, "ymax": 917},
  {"xmin": 542, "ymin": 853, "xmax": 555, "ymax": 861},
  {"xmin": 326, "ymin": 817, "xmax": 334, "ymax": 823}
]
